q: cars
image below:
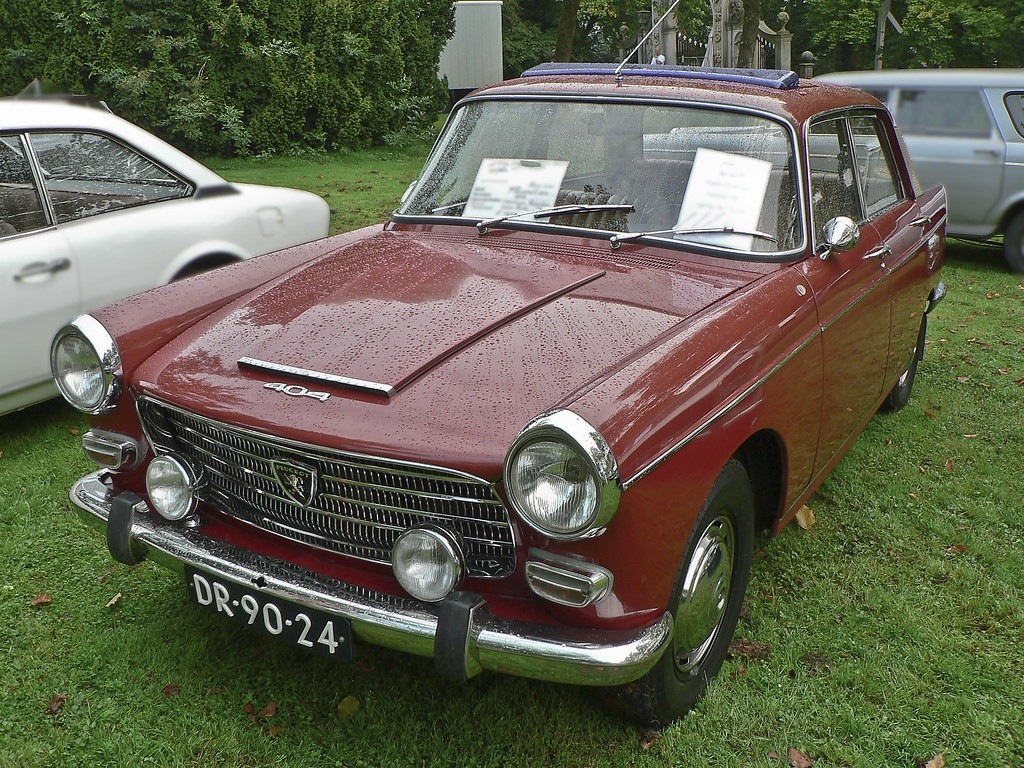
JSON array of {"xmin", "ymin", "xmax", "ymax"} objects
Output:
[
  {"xmin": 1, "ymin": 94, "xmax": 332, "ymax": 418},
  {"xmin": 642, "ymin": 66, "xmax": 1024, "ymax": 276},
  {"xmin": 48, "ymin": 63, "xmax": 953, "ymax": 728}
]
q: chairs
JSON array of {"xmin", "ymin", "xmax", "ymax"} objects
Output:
[{"xmin": 551, "ymin": 189, "xmax": 654, "ymax": 233}]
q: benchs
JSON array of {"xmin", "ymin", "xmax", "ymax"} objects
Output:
[
  {"xmin": 0, "ymin": 187, "xmax": 163, "ymax": 232},
  {"xmin": 620, "ymin": 158, "xmax": 853, "ymax": 238}
]
